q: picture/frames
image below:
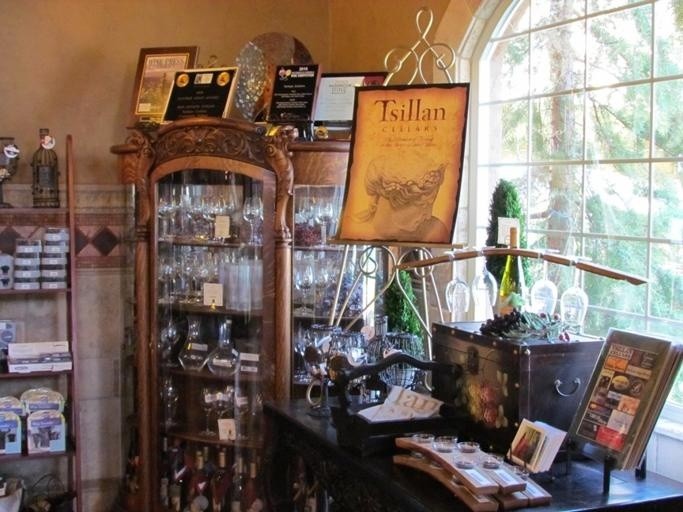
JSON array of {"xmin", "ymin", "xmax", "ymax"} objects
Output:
[
  {"xmin": 509, "ymin": 417, "xmax": 549, "ymax": 471},
  {"xmin": 310, "ymin": 71, "xmax": 392, "ymax": 127},
  {"xmin": 161, "ymin": 65, "xmax": 240, "ymax": 117},
  {"xmin": 266, "ymin": 62, "xmax": 323, "ymax": 124}
]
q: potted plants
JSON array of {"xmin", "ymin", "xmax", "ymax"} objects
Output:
[
  {"xmin": 484, "ymin": 180, "xmax": 529, "ymax": 332},
  {"xmin": 375, "ymin": 270, "xmax": 423, "ymax": 391}
]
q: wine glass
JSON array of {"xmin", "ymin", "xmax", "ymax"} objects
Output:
[
  {"xmin": 530, "ymin": 248, "xmax": 558, "ymax": 317},
  {"xmin": 295, "ymin": 184, "xmax": 337, "ymax": 245},
  {"xmin": 443, "ymin": 248, "xmax": 469, "ymax": 312},
  {"xmin": 159, "ymin": 172, "xmax": 263, "ymax": 245},
  {"xmin": 293, "ymin": 259, "xmax": 340, "ymax": 313},
  {"xmin": 154, "ymin": 377, "xmax": 234, "ymax": 440},
  {"xmin": 159, "ymin": 249, "xmax": 220, "ymax": 306},
  {"xmin": 558, "ymin": 255, "xmax": 591, "ymax": 324},
  {"xmin": 473, "ymin": 244, "xmax": 498, "ymax": 310}
]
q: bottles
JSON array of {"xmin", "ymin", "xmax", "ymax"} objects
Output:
[
  {"xmin": 155, "ymin": 434, "xmax": 263, "ymax": 512},
  {"xmin": 175, "ymin": 315, "xmax": 239, "ymax": 378},
  {"xmin": 30, "ymin": 126, "xmax": 60, "ymax": 208},
  {"xmin": 497, "ymin": 225, "xmax": 526, "ymax": 318}
]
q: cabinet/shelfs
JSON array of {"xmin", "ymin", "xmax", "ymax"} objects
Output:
[
  {"xmin": 0, "ymin": 126, "xmax": 83, "ymax": 512},
  {"xmin": 109, "ymin": 119, "xmax": 370, "ymax": 511}
]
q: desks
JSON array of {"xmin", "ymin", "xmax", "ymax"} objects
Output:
[{"xmin": 260, "ymin": 391, "xmax": 683, "ymax": 511}]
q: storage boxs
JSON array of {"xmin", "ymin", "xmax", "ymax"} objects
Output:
[{"xmin": 431, "ymin": 321, "xmax": 607, "ymax": 460}]
